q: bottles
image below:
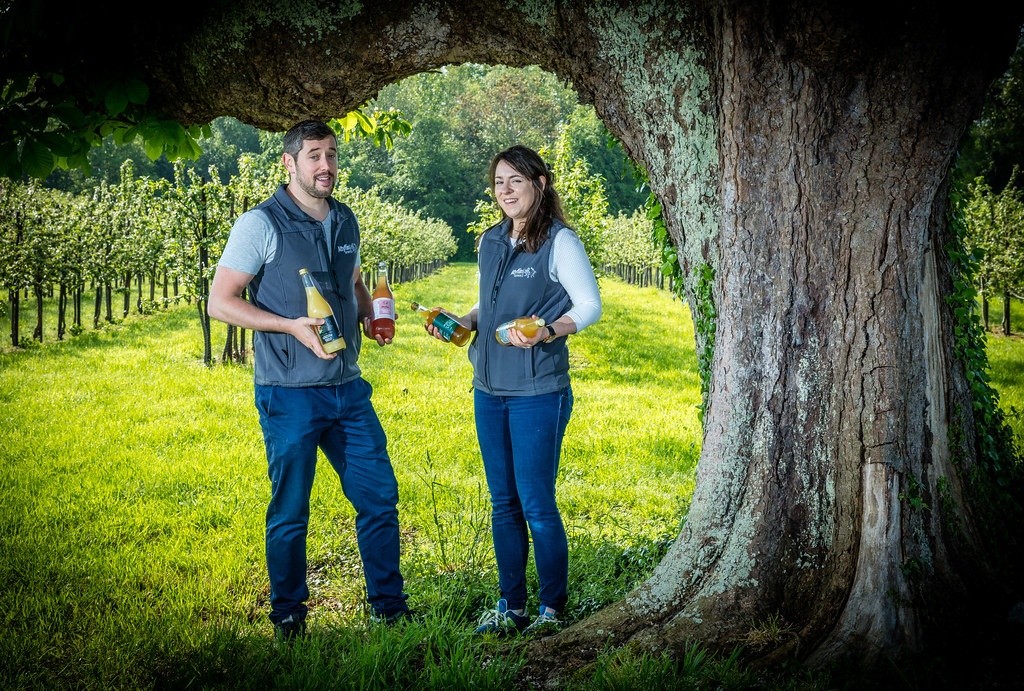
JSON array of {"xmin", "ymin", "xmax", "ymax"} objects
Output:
[
  {"xmin": 369, "ymin": 261, "xmax": 395, "ymax": 340},
  {"xmin": 410, "ymin": 301, "xmax": 471, "ymax": 347},
  {"xmin": 495, "ymin": 317, "xmax": 545, "ymax": 345},
  {"xmin": 299, "ymin": 270, "xmax": 347, "ymax": 353}
]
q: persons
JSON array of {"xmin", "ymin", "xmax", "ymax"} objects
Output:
[
  {"xmin": 424, "ymin": 145, "xmax": 601, "ymax": 639},
  {"xmin": 207, "ymin": 119, "xmax": 415, "ymax": 651}
]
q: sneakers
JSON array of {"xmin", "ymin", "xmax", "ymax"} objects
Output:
[
  {"xmin": 520, "ymin": 605, "xmax": 565, "ymax": 638},
  {"xmin": 472, "ymin": 598, "xmax": 533, "ymax": 640},
  {"xmin": 368, "ymin": 606, "xmax": 422, "ymax": 627},
  {"xmin": 274, "ymin": 613, "xmax": 306, "ymax": 641}
]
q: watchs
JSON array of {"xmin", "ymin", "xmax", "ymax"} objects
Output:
[{"xmin": 542, "ymin": 325, "xmax": 557, "ymax": 345}]
